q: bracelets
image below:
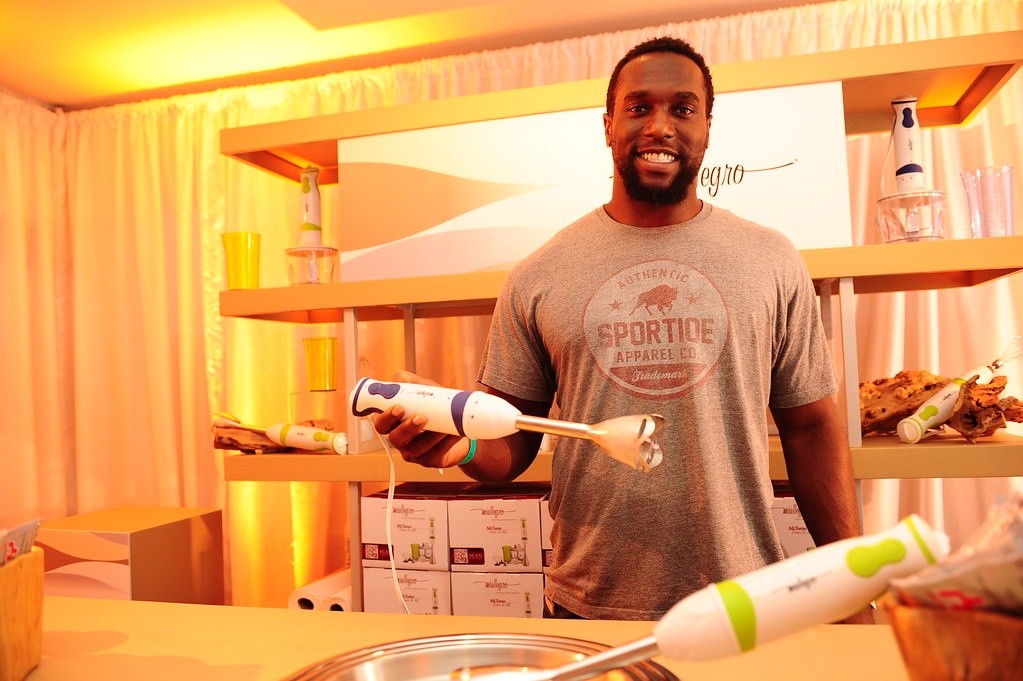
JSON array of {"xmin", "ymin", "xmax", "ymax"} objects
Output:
[{"xmin": 458, "ymin": 440, "xmax": 476, "ymax": 465}]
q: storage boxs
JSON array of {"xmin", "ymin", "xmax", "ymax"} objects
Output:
[{"xmin": 361, "ymin": 483, "xmax": 556, "ymax": 618}]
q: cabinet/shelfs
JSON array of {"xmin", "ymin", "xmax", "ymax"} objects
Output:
[{"xmin": 219, "ymin": 29, "xmax": 1023, "ymax": 614}]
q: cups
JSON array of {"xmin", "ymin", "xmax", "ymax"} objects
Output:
[
  {"xmin": 411, "ymin": 544, "xmax": 419, "ymax": 561},
  {"xmin": 302, "ymin": 337, "xmax": 338, "ymax": 391},
  {"xmin": 221, "ymin": 232, "xmax": 260, "ymax": 290},
  {"xmin": 960, "ymin": 166, "xmax": 1013, "ymax": 238},
  {"xmin": 502, "ymin": 545, "xmax": 512, "ymax": 562}
]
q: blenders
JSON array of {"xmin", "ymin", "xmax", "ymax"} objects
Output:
[
  {"xmin": 511, "ymin": 543, "xmax": 524, "ymax": 564},
  {"xmin": 418, "ymin": 542, "xmax": 431, "ymax": 564}
]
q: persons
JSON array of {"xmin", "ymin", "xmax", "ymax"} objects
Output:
[{"xmin": 375, "ymin": 36, "xmax": 876, "ymax": 624}]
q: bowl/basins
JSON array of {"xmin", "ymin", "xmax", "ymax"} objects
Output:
[
  {"xmin": 285, "ymin": 247, "xmax": 337, "ymax": 285},
  {"xmin": 285, "ymin": 633, "xmax": 680, "ymax": 681},
  {"xmin": 290, "ymin": 391, "xmax": 345, "ymax": 433}
]
